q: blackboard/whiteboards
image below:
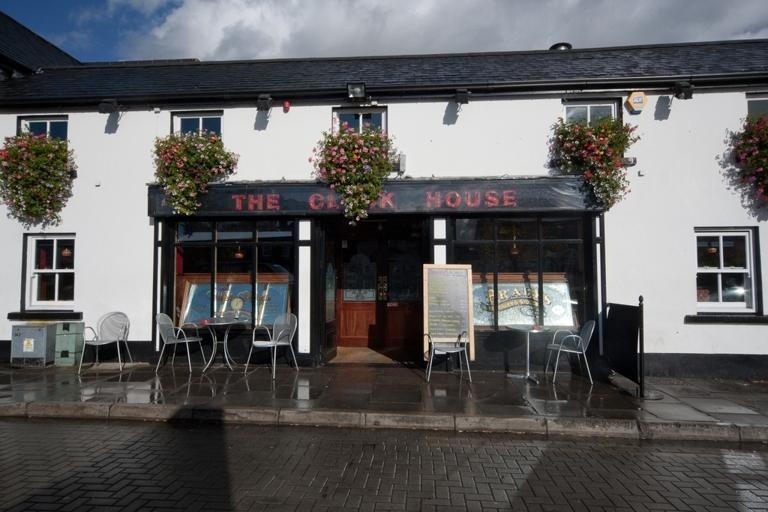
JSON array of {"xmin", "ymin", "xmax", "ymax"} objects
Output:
[{"xmin": 423, "ymin": 262, "xmax": 474, "ymax": 342}]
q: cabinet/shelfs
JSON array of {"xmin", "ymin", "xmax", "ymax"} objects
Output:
[{"xmin": 54, "ymin": 322, "xmax": 85, "ymax": 367}]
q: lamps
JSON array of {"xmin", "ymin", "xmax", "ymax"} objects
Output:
[
  {"xmin": 672, "ymin": 82, "xmax": 693, "ymax": 100},
  {"xmin": 256, "ymin": 94, "xmax": 273, "ymax": 111},
  {"xmin": 99, "ymin": 99, "xmax": 126, "ymax": 114},
  {"xmin": 347, "ymin": 82, "xmax": 368, "ymax": 103},
  {"xmin": 455, "ymin": 89, "xmax": 469, "ymax": 104}
]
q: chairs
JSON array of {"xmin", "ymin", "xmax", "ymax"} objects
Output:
[
  {"xmin": 78, "ymin": 313, "xmax": 133, "ymax": 375},
  {"xmin": 424, "ymin": 331, "xmax": 472, "ymax": 383},
  {"xmin": 245, "ymin": 313, "xmax": 298, "ymax": 379},
  {"xmin": 155, "ymin": 313, "xmax": 206, "ymax": 373},
  {"xmin": 546, "ymin": 320, "xmax": 595, "ymax": 385}
]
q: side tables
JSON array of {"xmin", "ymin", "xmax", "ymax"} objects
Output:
[{"xmin": 505, "ymin": 324, "xmax": 550, "ymax": 385}]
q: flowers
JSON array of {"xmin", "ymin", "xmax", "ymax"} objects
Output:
[
  {"xmin": 0, "ymin": 132, "xmax": 77, "ymax": 228},
  {"xmin": 549, "ymin": 118, "xmax": 638, "ymax": 211},
  {"xmin": 728, "ymin": 114, "xmax": 768, "ymax": 208},
  {"xmin": 151, "ymin": 132, "xmax": 238, "ymax": 216},
  {"xmin": 308, "ymin": 122, "xmax": 400, "ymax": 227}
]
